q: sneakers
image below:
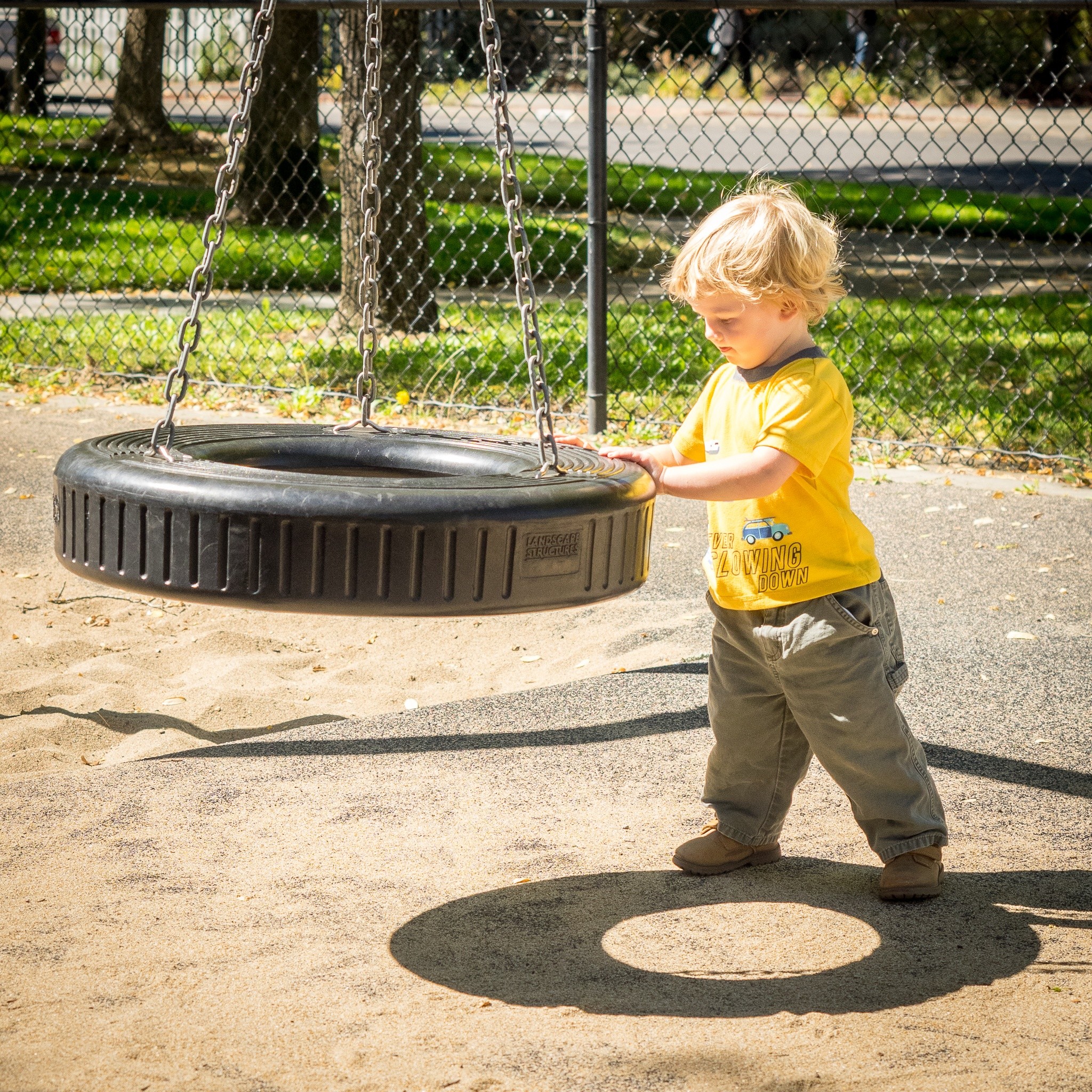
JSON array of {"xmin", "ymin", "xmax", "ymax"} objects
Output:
[
  {"xmin": 672, "ymin": 822, "xmax": 781, "ymax": 874},
  {"xmin": 880, "ymin": 845, "xmax": 944, "ymax": 900}
]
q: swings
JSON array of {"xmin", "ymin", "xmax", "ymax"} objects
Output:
[{"xmin": 54, "ymin": 0, "xmax": 659, "ymax": 618}]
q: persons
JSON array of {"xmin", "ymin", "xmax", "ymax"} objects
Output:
[
  {"xmin": 697, "ymin": 7, "xmax": 766, "ymax": 95},
  {"xmin": 848, "ymin": 8, "xmax": 877, "ymax": 85},
  {"xmin": 544, "ymin": 179, "xmax": 951, "ymax": 899}
]
